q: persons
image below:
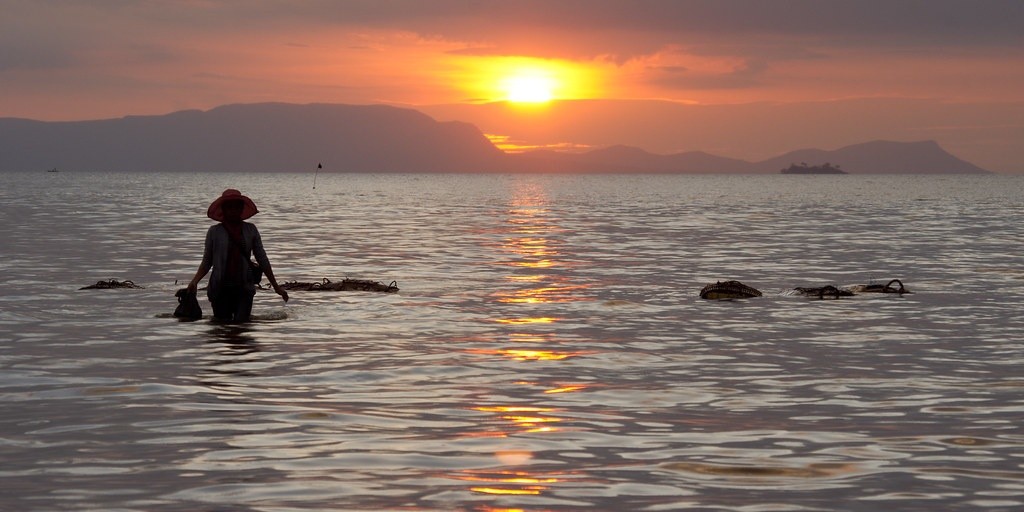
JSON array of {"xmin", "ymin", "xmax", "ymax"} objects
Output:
[{"xmin": 186, "ymin": 190, "xmax": 290, "ymax": 324}]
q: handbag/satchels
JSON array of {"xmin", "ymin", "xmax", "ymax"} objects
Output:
[
  {"xmin": 173, "ymin": 286, "xmax": 203, "ymax": 323},
  {"xmin": 250, "ymin": 263, "xmax": 262, "ymax": 284}
]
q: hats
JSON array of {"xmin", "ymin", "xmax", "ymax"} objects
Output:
[{"xmin": 206, "ymin": 189, "xmax": 259, "ymax": 223}]
